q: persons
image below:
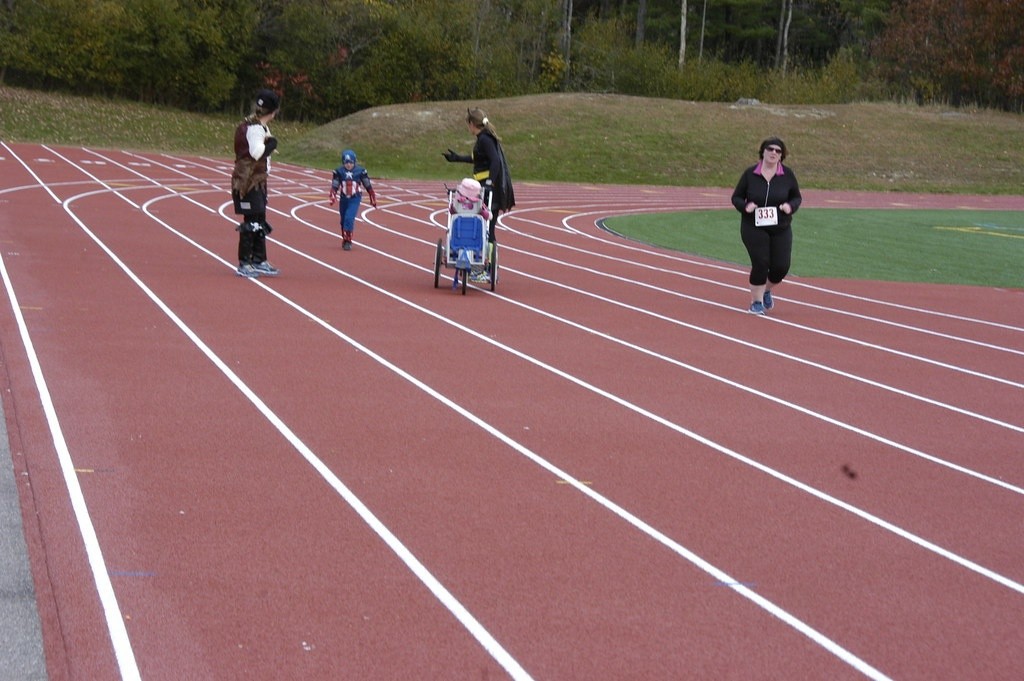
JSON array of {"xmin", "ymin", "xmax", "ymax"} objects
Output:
[
  {"xmin": 440, "ymin": 106, "xmax": 515, "ymax": 282},
  {"xmin": 729, "ymin": 137, "xmax": 802, "ymax": 315},
  {"xmin": 329, "ymin": 151, "xmax": 377, "ymax": 250},
  {"xmin": 449, "ymin": 179, "xmax": 493, "ymax": 222},
  {"xmin": 231, "ymin": 88, "xmax": 281, "ymax": 278}
]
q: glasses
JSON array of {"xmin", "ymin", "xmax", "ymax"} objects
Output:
[{"xmin": 765, "ymin": 146, "xmax": 783, "ymax": 153}]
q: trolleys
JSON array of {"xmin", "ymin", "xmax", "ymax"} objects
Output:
[{"xmin": 433, "ymin": 183, "xmax": 500, "ymax": 296}]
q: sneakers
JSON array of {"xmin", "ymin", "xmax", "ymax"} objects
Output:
[
  {"xmin": 235, "ymin": 265, "xmax": 259, "ymax": 277},
  {"xmin": 471, "ymin": 271, "xmax": 500, "ymax": 284},
  {"xmin": 763, "ymin": 290, "xmax": 774, "ymax": 310},
  {"xmin": 468, "ymin": 267, "xmax": 484, "ymax": 280},
  {"xmin": 749, "ymin": 301, "xmax": 765, "ymax": 315},
  {"xmin": 246, "ymin": 261, "xmax": 280, "ymax": 275}
]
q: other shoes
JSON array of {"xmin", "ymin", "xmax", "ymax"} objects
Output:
[{"xmin": 342, "ymin": 241, "xmax": 352, "ymax": 250}]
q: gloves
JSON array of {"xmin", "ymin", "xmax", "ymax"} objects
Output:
[
  {"xmin": 482, "ymin": 182, "xmax": 494, "ymax": 191},
  {"xmin": 442, "ymin": 148, "xmax": 459, "ymax": 162}
]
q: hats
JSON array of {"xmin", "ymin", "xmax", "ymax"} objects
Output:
[
  {"xmin": 459, "ymin": 178, "xmax": 481, "ymax": 201},
  {"xmin": 342, "ymin": 150, "xmax": 356, "ymax": 164},
  {"xmin": 256, "ymin": 91, "xmax": 279, "ymax": 109}
]
